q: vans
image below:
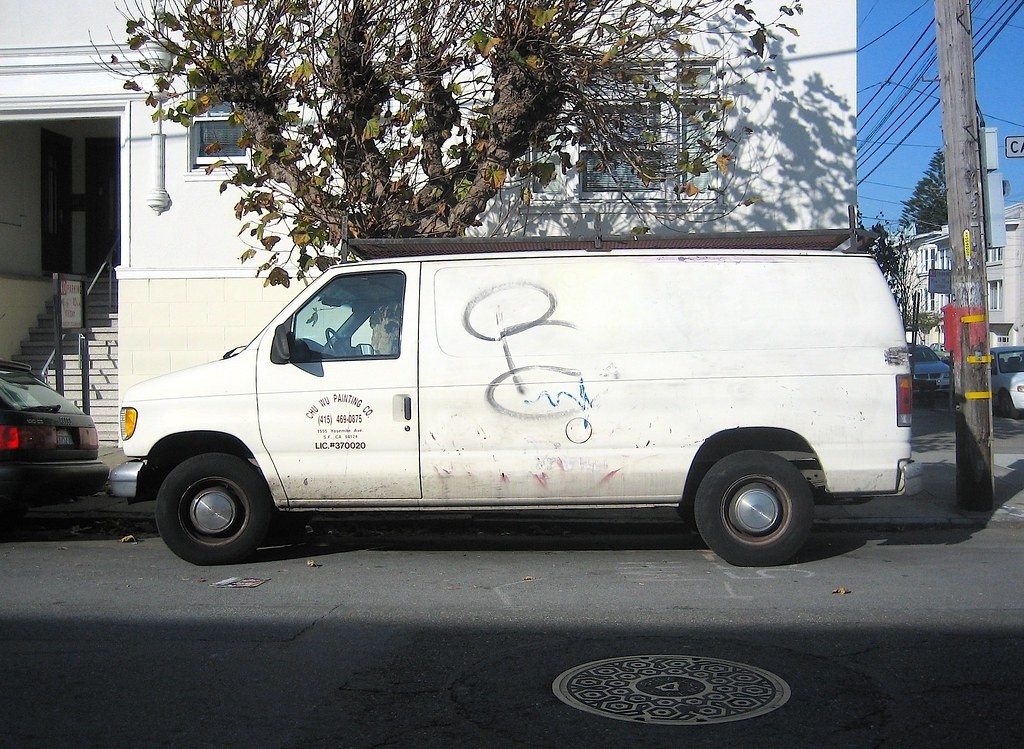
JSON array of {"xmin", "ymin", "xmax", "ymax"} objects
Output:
[{"xmin": 105, "ymin": 248, "xmax": 912, "ymax": 567}]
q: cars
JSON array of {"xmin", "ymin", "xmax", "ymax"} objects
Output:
[
  {"xmin": 990, "ymin": 345, "xmax": 1024, "ymax": 416},
  {"xmin": 906, "ymin": 343, "xmax": 951, "ymax": 391},
  {"xmin": 0, "ymin": 362, "xmax": 110, "ymax": 530}
]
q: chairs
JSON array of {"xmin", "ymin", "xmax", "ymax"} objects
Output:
[
  {"xmin": 992, "ymin": 358, "xmax": 1005, "ymax": 370},
  {"xmin": 1008, "ymin": 356, "xmax": 1020, "ymax": 370}
]
